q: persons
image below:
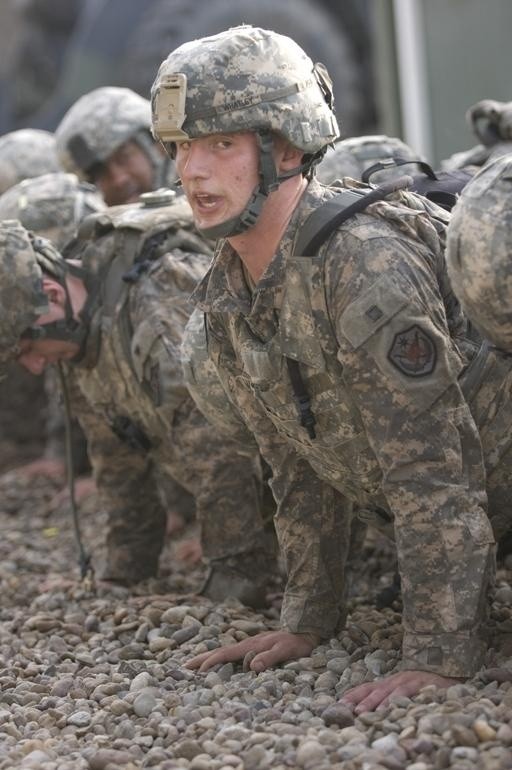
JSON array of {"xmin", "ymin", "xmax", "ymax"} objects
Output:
[{"xmin": 1, "ymin": 26, "xmax": 512, "ymax": 715}]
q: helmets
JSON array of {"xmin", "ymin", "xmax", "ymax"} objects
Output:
[
  {"xmin": 314, "ymin": 133, "xmax": 423, "ymax": 187},
  {"xmin": 51, "ymin": 86, "xmax": 185, "ymax": 198},
  {"xmin": 0, "ymin": 218, "xmax": 50, "ymax": 376},
  {"xmin": 443, "ymin": 152, "xmax": 512, "ymax": 352},
  {"xmin": 0, "ymin": 126, "xmax": 66, "ymax": 196},
  {"xmin": 0, "ymin": 170, "xmax": 111, "ymax": 253},
  {"xmin": 149, "ymin": 24, "xmax": 341, "ymax": 154}
]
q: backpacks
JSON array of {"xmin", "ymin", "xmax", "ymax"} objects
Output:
[{"xmin": 283, "ymin": 154, "xmax": 482, "ymax": 442}]
